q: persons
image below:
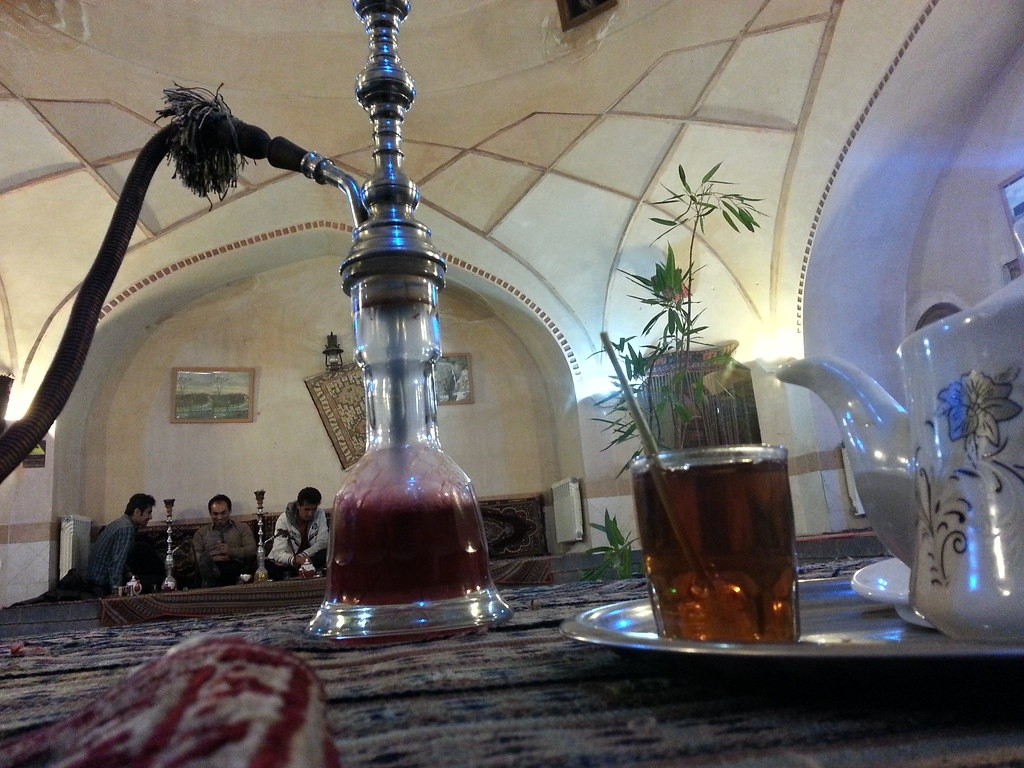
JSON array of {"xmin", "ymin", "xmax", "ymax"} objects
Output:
[
  {"xmin": 188, "ymin": 494, "xmax": 257, "ymax": 589},
  {"xmin": 85, "ymin": 493, "xmax": 160, "ymax": 598},
  {"xmin": 263, "ymin": 487, "xmax": 332, "ymax": 580}
]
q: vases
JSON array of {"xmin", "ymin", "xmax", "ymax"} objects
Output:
[{"xmin": 638, "ymin": 342, "xmax": 763, "ymax": 451}]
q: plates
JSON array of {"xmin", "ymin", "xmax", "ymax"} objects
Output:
[{"xmin": 850, "ymin": 558, "xmax": 935, "ymax": 627}]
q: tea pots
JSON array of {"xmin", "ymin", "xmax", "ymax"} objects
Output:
[
  {"xmin": 298, "ymin": 558, "xmax": 315, "ymax": 578},
  {"xmin": 127, "ymin": 575, "xmax": 142, "ymax": 596},
  {"xmin": 775, "ymin": 214, "xmax": 1024, "ymax": 642}
]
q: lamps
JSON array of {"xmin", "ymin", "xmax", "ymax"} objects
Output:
[{"xmin": 323, "ymin": 331, "xmax": 343, "ymax": 371}]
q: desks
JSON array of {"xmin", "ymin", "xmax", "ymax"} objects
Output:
[{"xmin": 0, "ymin": 555, "xmax": 1024, "ymax": 768}]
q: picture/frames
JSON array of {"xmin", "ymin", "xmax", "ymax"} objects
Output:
[
  {"xmin": 433, "ymin": 352, "xmax": 475, "ymax": 405},
  {"xmin": 169, "ymin": 367, "xmax": 255, "ymax": 423}
]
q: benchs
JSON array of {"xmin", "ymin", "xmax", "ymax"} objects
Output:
[{"xmin": 1, "ymin": 491, "xmax": 552, "ymax": 639}]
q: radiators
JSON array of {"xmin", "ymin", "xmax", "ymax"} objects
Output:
[
  {"xmin": 60, "ymin": 514, "xmax": 92, "ymax": 580},
  {"xmin": 552, "ymin": 477, "xmax": 583, "ymax": 543}
]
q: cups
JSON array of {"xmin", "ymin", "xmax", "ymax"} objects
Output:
[{"xmin": 630, "ymin": 444, "xmax": 801, "ymax": 644}]
